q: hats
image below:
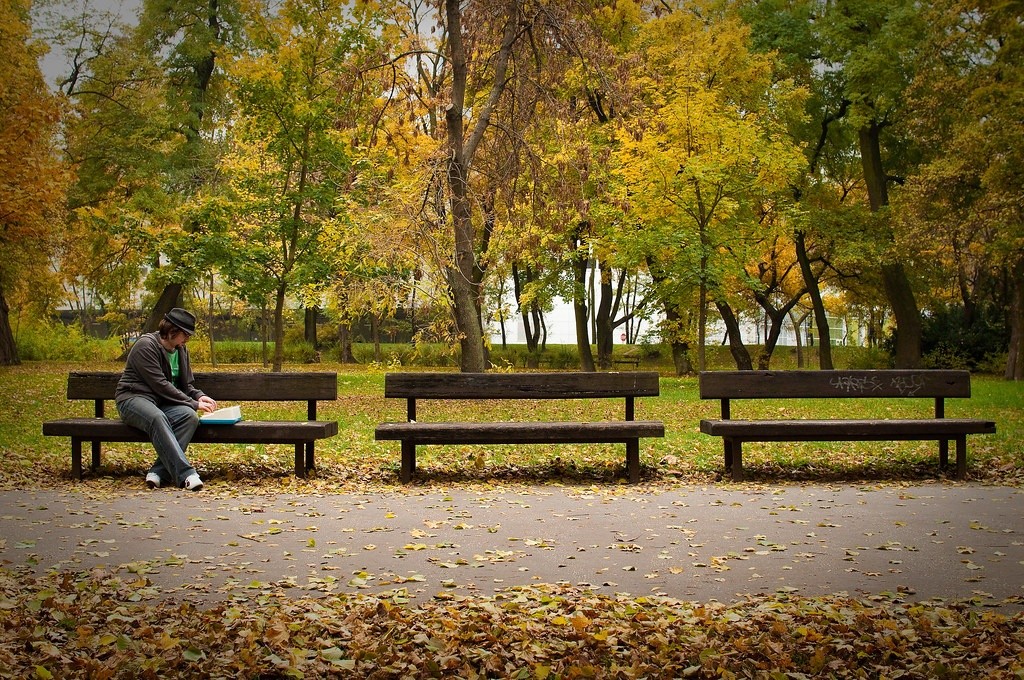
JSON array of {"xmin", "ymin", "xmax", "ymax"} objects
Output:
[{"xmin": 165, "ymin": 308, "xmax": 196, "ymax": 334}]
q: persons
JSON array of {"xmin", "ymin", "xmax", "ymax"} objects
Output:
[{"xmin": 115, "ymin": 309, "xmax": 217, "ymax": 489}]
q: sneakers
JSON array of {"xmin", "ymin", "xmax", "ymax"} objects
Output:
[
  {"xmin": 146, "ymin": 473, "xmax": 160, "ymax": 487},
  {"xmin": 185, "ymin": 473, "xmax": 203, "ymax": 490}
]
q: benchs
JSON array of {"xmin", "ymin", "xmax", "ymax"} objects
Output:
[
  {"xmin": 696, "ymin": 370, "xmax": 999, "ymax": 483},
  {"xmin": 41, "ymin": 372, "xmax": 338, "ymax": 480},
  {"xmin": 375, "ymin": 369, "xmax": 665, "ymax": 482}
]
